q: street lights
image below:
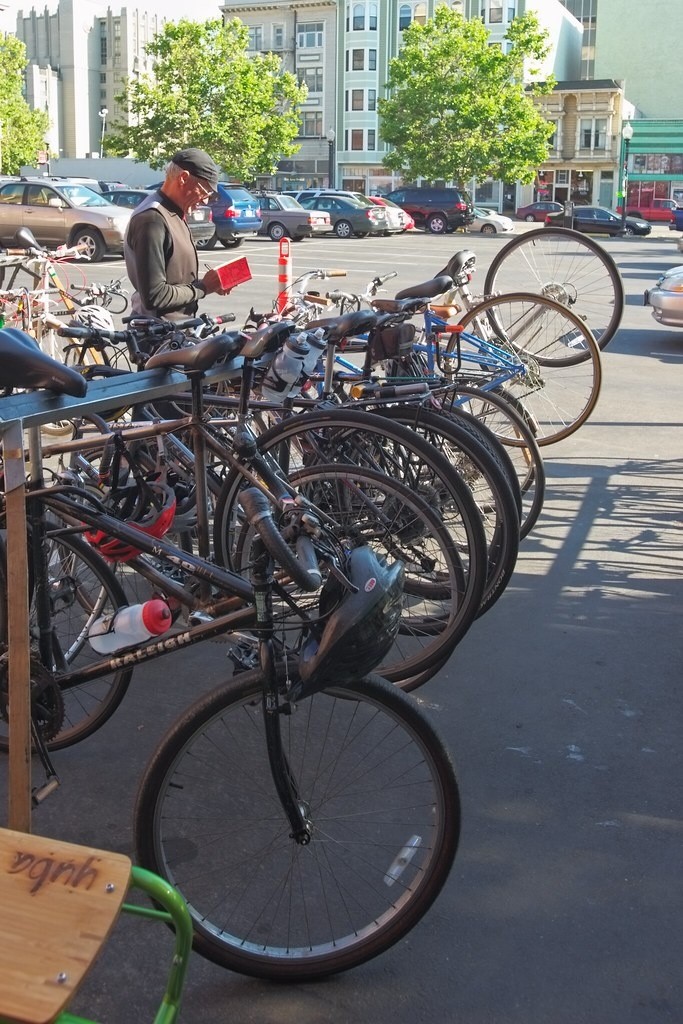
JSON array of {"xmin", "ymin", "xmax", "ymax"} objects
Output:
[
  {"xmin": 325, "ymin": 130, "xmax": 339, "ymax": 189},
  {"xmin": 620, "ymin": 124, "xmax": 633, "ymax": 237}
]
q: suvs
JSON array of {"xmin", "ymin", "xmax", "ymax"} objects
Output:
[
  {"xmin": 0, "ymin": 179, "xmax": 133, "ymax": 265},
  {"xmin": 386, "ymin": 188, "xmax": 473, "ymax": 235}
]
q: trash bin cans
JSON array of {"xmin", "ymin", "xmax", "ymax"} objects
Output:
[{"xmin": 672, "ymin": 210, "xmax": 683, "ymax": 231}]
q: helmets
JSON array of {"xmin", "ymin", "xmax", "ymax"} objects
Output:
[
  {"xmin": 288, "ymin": 548, "xmax": 406, "ymax": 700},
  {"xmin": 68, "ymin": 305, "xmax": 115, "ymax": 333},
  {"xmin": 83, "ymin": 481, "xmax": 177, "ymax": 562}
]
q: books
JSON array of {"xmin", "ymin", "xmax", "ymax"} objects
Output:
[{"xmin": 214, "ymin": 256, "xmax": 252, "ymax": 290}]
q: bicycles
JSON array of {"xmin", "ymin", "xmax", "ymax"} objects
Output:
[
  {"xmin": 0, "ymin": 326, "xmax": 459, "ymax": 984},
  {"xmin": 0, "ymin": 230, "xmax": 626, "ymax": 706}
]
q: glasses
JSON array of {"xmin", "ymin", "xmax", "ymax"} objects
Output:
[{"xmin": 188, "ymin": 174, "xmax": 213, "ymax": 200}]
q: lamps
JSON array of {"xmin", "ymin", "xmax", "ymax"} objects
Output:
[
  {"xmin": 540, "ymin": 171, "xmax": 544, "ymax": 176},
  {"xmin": 579, "ymin": 171, "xmax": 583, "ymax": 178}
]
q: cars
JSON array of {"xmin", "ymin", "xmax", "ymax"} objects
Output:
[
  {"xmin": 647, "ymin": 262, "xmax": 682, "ymax": 328},
  {"xmin": 96, "ymin": 180, "xmax": 413, "ymax": 250},
  {"xmin": 546, "ymin": 206, "xmax": 652, "ymax": 237},
  {"xmin": 516, "ymin": 201, "xmax": 564, "ymax": 222},
  {"xmin": 471, "ymin": 208, "xmax": 514, "ymax": 234}
]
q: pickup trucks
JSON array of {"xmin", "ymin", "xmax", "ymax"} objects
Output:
[
  {"xmin": 669, "ymin": 207, "xmax": 683, "ymax": 231},
  {"xmin": 616, "ymin": 198, "xmax": 680, "ymax": 221}
]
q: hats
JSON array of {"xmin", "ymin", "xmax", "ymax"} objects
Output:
[{"xmin": 171, "ymin": 148, "xmax": 219, "ymax": 195}]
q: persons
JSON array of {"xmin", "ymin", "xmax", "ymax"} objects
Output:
[{"xmin": 123, "ymin": 148, "xmax": 231, "ymax": 421}]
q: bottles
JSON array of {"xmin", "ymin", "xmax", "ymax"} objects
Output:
[
  {"xmin": 89, "ymin": 600, "xmax": 171, "ymax": 655},
  {"xmin": 261, "ymin": 332, "xmax": 310, "ymax": 402},
  {"xmin": 286, "ymin": 328, "xmax": 327, "ymax": 398}
]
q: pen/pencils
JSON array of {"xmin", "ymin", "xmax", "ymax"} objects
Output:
[{"xmin": 204, "ymin": 263, "xmax": 212, "ymax": 271}]
